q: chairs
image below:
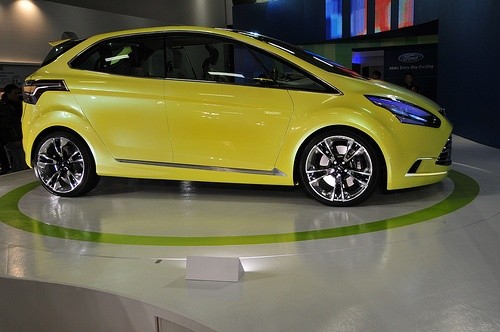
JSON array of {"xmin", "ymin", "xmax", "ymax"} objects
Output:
[
  {"xmin": 95, "ymin": 48, "xmax": 132, "ymax": 74},
  {"xmin": 202, "ymin": 45, "xmax": 226, "ymax": 82},
  {"xmin": 168, "ymin": 50, "xmax": 190, "ymax": 78}
]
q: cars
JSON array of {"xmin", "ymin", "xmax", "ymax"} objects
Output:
[{"xmin": 19, "ymin": 25, "xmax": 454, "ymax": 208}]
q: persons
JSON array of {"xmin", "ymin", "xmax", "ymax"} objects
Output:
[
  {"xmin": 0, "ymin": 83, "xmax": 27, "ymax": 173},
  {"xmin": 371, "ymin": 71, "xmax": 383, "ymax": 79},
  {"xmin": 401, "ymin": 73, "xmax": 420, "ymax": 94}
]
